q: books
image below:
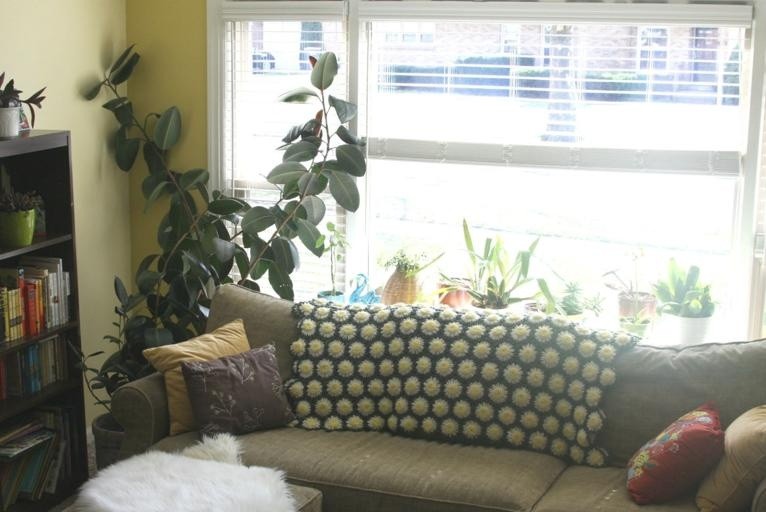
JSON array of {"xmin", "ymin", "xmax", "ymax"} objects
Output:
[{"xmin": 0, "ymin": 254, "xmax": 71, "ymax": 512}]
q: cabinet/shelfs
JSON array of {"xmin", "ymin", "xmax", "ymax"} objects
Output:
[{"xmin": 1, "ymin": 129, "xmax": 90, "ymax": 512}]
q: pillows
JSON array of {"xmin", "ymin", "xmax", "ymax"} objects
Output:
[
  {"xmin": 626, "ymin": 399, "xmax": 725, "ymax": 505},
  {"xmin": 180, "ymin": 341, "xmax": 299, "ymax": 438},
  {"xmin": 141, "ymin": 319, "xmax": 251, "ymax": 435},
  {"xmin": 695, "ymin": 404, "xmax": 766, "ymax": 512}
]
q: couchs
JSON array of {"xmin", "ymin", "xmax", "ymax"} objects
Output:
[{"xmin": 108, "ymin": 284, "xmax": 766, "ymax": 511}]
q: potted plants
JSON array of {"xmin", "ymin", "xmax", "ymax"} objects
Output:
[
  {"xmin": 1, "ymin": 72, "xmax": 46, "ymax": 139},
  {"xmin": 61, "ymin": 42, "xmax": 368, "ymax": 472},
  {"xmin": 3, "ymin": 190, "xmax": 38, "ymax": 247}
]
